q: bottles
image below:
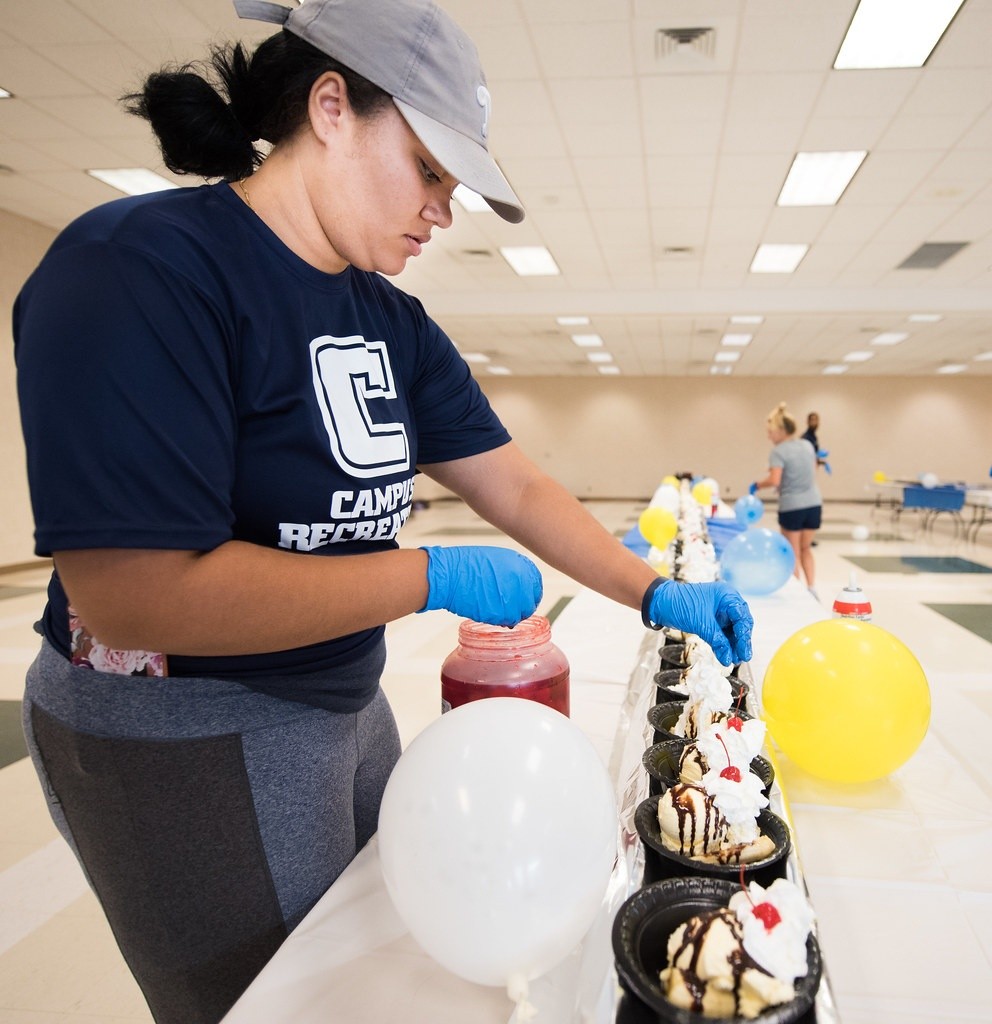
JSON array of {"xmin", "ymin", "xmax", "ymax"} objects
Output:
[{"xmin": 832, "ymin": 587, "xmax": 873, "ymax": 625}]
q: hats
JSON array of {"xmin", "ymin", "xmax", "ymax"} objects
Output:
[{"xmin": 233, "ymin": 0, "xmax": 525, "ymax": 224}]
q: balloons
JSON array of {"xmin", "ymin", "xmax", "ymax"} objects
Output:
[
  {"xmin": 377, "ymin": 698, "xmax": 622, "ymax": 1022},
  {"xmin": 637, "ymin": 473, "xmax": 795, "ymax": 599},
  {"xmin": 762, "ymin": 619, "xmax": 931, "ymax": 788},
  {"xmin": 873, "ymin": 470, "xmax": 938, "ymax": 490}
]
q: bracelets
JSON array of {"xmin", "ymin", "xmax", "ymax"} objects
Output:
[{"xmin": 641, "ymin": 576, "xmax": 670, "ymax": 630}]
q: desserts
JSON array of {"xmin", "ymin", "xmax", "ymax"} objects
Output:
[{"xmin": 611, "ymin": 505, "xmax": 822, "ymax": 1024}]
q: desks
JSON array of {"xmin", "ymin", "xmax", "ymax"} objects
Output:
[{"xmin": 218, "ymin": 497, "xmax": 992, "ymax": 1024}]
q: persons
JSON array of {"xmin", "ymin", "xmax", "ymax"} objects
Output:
[
  {"xmin": 750, "ymin": 401, "xmax": 824, "ymax": 605},
  {"xmin": 799, "ymin": 413, "xmax": 831, "ymax": 546},
  {"xmin": 13, "ymin": 0, "xmax": 752, "ymax": 1024}
]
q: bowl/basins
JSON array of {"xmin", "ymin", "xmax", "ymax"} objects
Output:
[
  {"xmin": 664, "ymin": 627, "xmax": 687, "ymax": 647},
  {"xmin": 673, "ymin": 539, "xmax": 718, "ymax": 585},
  {"xmin": 634, "ymin": 795, "xmax": 791, "ymax": 890},
  {"xmin": 611, "ymin": 877, "xmax": 823, "ymax": 1024},
  {"xmin": 654, "ymin": 670, "xmax": 750, "ymax": 713},
  {"xmin": 642, "ymin": 739, "xmax": 775, "ymax": 810},
  {"xmin": 659, "ymin": 644, "xmax": 741, "ymax": 680},
  {"xmin": 647, "ymin": 700, "xmax": 756, "ymax": 746}
]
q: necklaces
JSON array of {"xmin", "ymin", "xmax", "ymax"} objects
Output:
[{"xmin": 239, "ymin": 177, "xmax": 255, "ymax": 208}]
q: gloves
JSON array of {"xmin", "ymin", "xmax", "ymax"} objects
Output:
[
  {"xmin": 825, "ymin": 464, "xmax": 832, "ymax": 474},
  {"xmin": 415, "ymin": 546, "xmax": 543, "ymax": 629},
  {"xmin": 649, "ymin": 580, "xmax": 754, "ymax": 667},
  {"xmin": 750, "ymin": 483, "xmax": 757, "ymax": 495},
  {"xmin": 818, "ymin": 450, "xmax": 829, "ymax": 458}
]
